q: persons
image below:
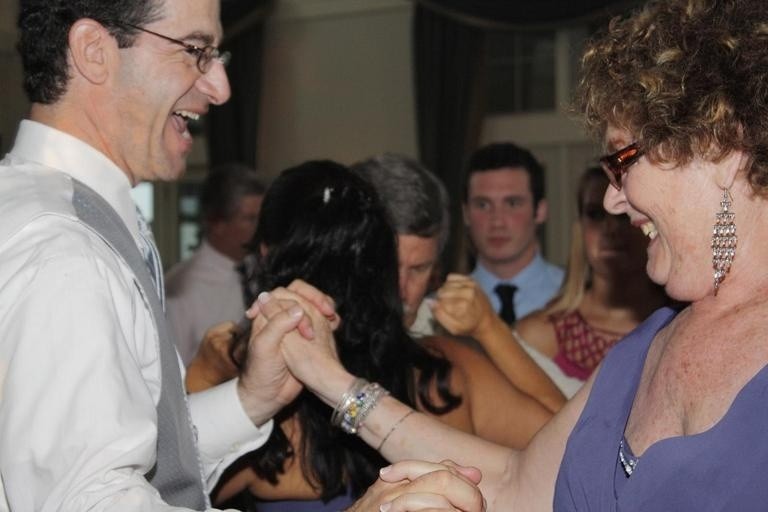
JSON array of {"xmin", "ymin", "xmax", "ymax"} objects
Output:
[
  {"xmin": 1, "ymin": 1, "xmax": 489, "ymax": 512},
  {"xmin": 242, "ymin": 1, "xmax": 767, "ymax": 512}
]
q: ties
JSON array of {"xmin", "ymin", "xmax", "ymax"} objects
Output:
[
  {"xmin": 235, "ymin": 263, "xmax": 254, "ymax": 310},
  {"xmin": 494, "ymin": 284, "xmax": 518, "ymax": 324}
]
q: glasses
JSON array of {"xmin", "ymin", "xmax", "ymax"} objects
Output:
[
  {"xmin": 598, "ymin": 127, "xmax": 668, "ymax": 193},
  {"xmin": 111, "ymin": 19, "xmax": 233, "ymax": 75}
]
